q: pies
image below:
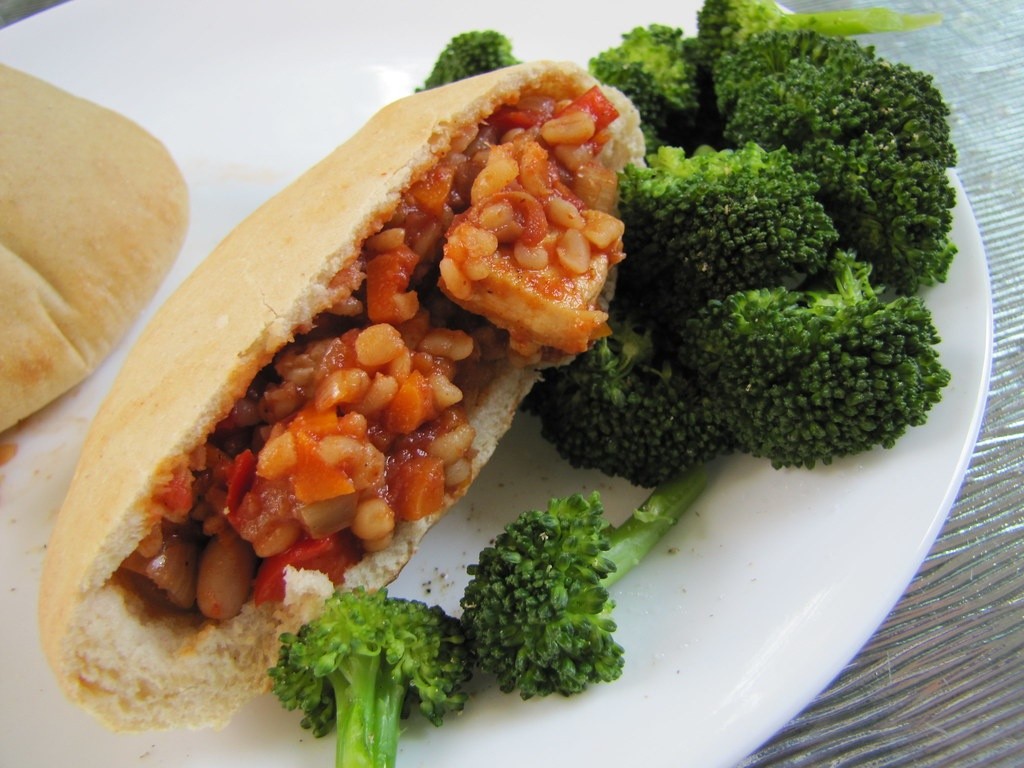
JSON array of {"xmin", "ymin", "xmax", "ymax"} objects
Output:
[
  {"xmin": 0, "ymin": 61, "xmax": 187, "ymax": 437},
  {"xmin": 40, "ymin": 61, "xmax": 645, "ymax": 735}
]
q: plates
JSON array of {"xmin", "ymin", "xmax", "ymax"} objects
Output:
[{"xmin": 0, "ymin": 1, "xmax": 992, "ymax": 768}]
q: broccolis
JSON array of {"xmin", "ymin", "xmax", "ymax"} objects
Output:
[{"xmin": 263, "ymin": 0, "xmax": 956, "ymax": 768}]
